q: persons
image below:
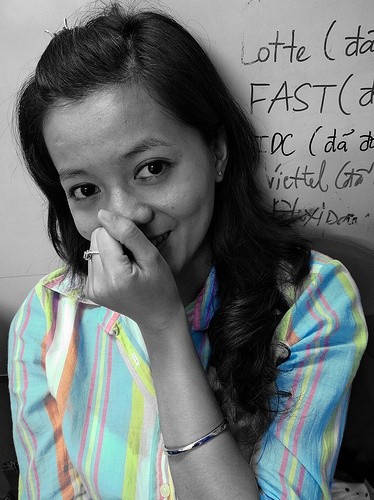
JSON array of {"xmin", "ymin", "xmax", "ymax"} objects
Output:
[{"xmin": 0, "ymin": 6, "xmax": 367, "ymax": 500}]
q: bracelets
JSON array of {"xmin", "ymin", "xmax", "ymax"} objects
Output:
[{"xmin": 153, "ymin": 415, "xmax": 231, "ymax": 462}]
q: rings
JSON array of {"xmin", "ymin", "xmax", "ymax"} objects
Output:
[{"xmin": 80, "ymin": 249, "xmax": 101, "ymax": 261}]
